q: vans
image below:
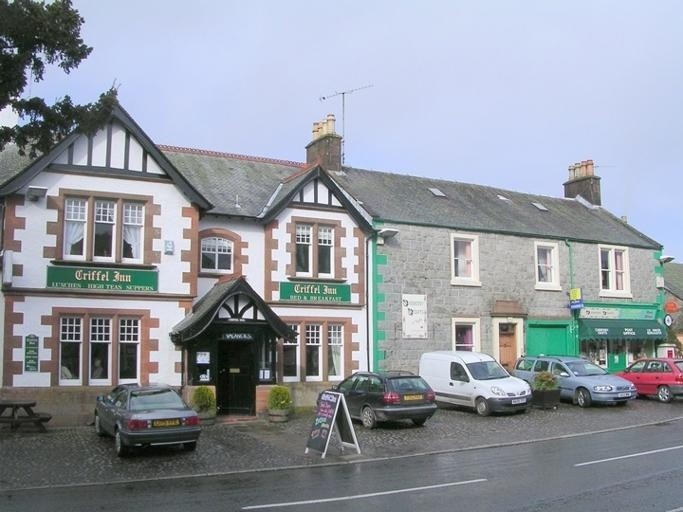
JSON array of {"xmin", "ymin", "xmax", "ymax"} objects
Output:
[
  {"xmin": 417, "ymin": 348, "xmax": 533, "ymax": 420},
  {"xmin": 316, "ymin": 368, "xmax": 438, "ymax": 431},
  {"xmin": 513, "ymin": 353, "xmax": 640, "ymax": 410}
]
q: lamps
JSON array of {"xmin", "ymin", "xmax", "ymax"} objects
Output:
[
  {"xmin": 27, "ymin": 183, "xmax": 49, "ymax": 201},
  {"xmin": 377, "ymin": 227, "xmax": 399, "ymax": 245}
]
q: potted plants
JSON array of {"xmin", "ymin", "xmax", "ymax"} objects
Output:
[
  {"xmin": 189, "ymin": 386, "xmax": 216, "ymax": 429},
  {"xmin": 266, "ymin": 383, "xmax": 290, "ymax": 422}
]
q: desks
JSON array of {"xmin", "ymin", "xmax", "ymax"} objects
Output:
[{"xmin": 0, "ymin": 398, "xmax": 48, "ymax": 434}]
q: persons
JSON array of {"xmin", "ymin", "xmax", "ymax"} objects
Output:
[{"xmin": 91, "ymin": 359, "xmax": 102, "ymax": 378}]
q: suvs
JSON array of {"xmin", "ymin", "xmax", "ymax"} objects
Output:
[{"xmin": 613, "ymin": 356, "xmax": 683, "ymax": 402}]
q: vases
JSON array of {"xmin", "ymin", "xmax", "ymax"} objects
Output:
[{"xmin": 530, "ymin": 389, "xmax": 559, "ymax": 411}]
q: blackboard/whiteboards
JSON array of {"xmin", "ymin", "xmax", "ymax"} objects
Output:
[{"xmin": 306, "ymin": 391, "xmax": 342, "ymax": 455}]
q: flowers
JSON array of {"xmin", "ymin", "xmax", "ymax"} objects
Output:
[{"xmin": 528, "ymin": 370, "xmax": 561, "ymax": 392}]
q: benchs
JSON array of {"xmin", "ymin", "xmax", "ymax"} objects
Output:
[{"xmin": 0, "ymin": 412, "xmax": 52, "ymax": 425}]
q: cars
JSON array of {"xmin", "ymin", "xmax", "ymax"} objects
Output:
[{"xmin": 93, "ymin": 382, "xmax": 201, "ymax": 457}]
